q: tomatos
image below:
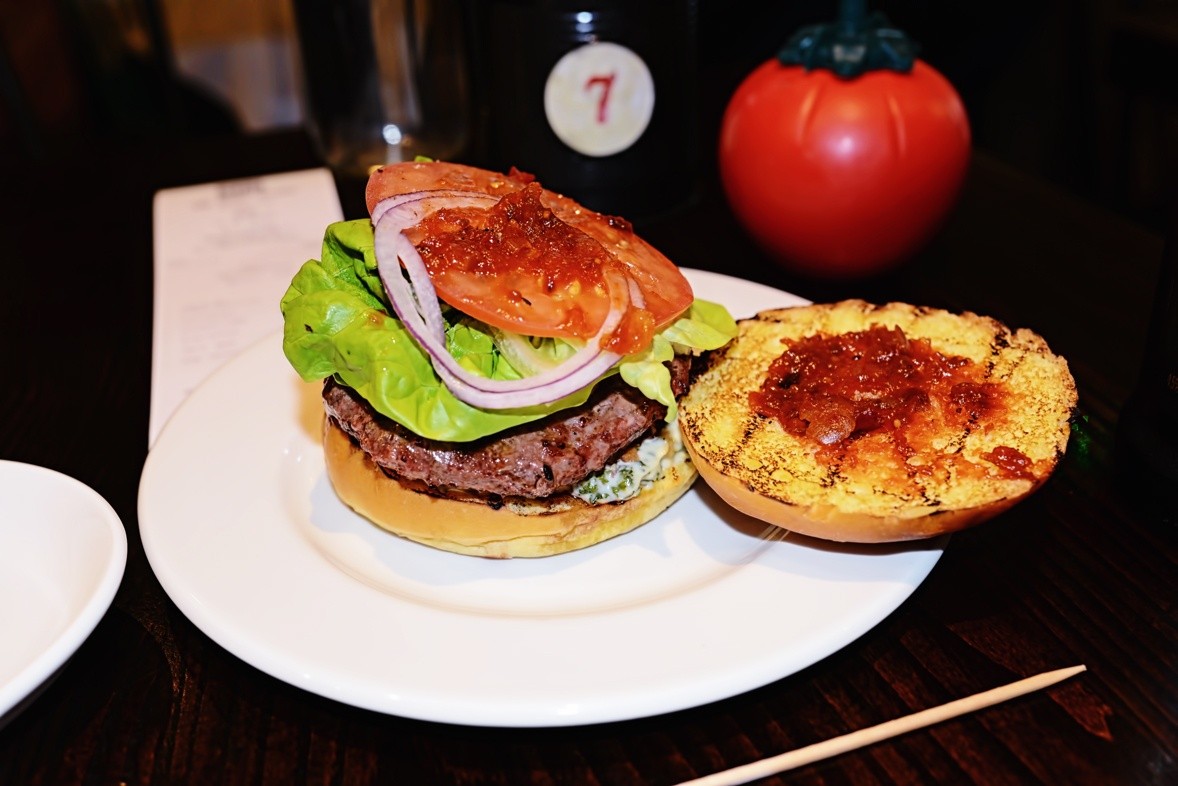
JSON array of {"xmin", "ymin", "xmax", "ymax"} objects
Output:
[{"xmin": 723, "ymin": 17, "xmax": 974, "ymax": 267}]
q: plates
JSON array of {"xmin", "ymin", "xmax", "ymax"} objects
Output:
[
  {"xmin": 136, "ymin": 268, "xmax": 950, "ymax": 729},
  {"xmin": 1, "ymin": 457, "xmax": 126, "ymax": 717}
]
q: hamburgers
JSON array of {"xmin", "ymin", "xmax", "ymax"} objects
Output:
[{"xmin": 285, "ymin": 146, "xmax": 1083, "ymax": 560}]
